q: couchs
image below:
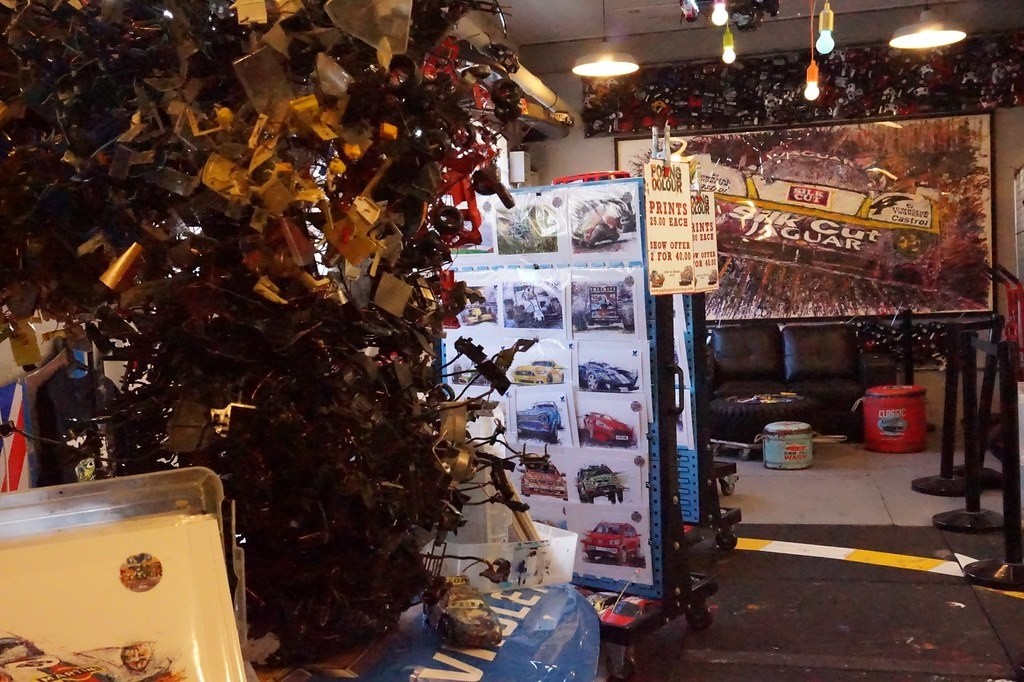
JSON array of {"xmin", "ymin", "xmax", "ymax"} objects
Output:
[{"xmin": 706, "ymin": 324, "xmax": 885, "ymax": 449}]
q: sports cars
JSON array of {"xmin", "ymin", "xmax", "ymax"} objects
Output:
[
  {"xmin": 514, "ymin": 360, "xmax": 565, "ymax": 386},
  {"xmin": 578, "ymin": 361, "xmax": 638, "ymax": 391}
]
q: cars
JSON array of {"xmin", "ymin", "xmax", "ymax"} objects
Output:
[
  {"xmin": 584, "ymin": 413, "xmax": 637, "ymax": 446},
  {"xmin": 521, "ymin": 464, "xmax": 568, "ymax": 501},
  {"xmin": 516, "ymin": 401, "xmax": 562, "ymax": 442},
  {"xmin": 423, "ymin": 575, "xmax": 501, "ymax": 647},
  {"xmin": 596, "ymin": 596, "xmax": 654, "ymax": 627},
  {"xmin": 587, "ymin": 592, "xmax": 620, "ymax": 611}
]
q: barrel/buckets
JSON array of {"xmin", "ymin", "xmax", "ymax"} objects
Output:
[
  {"xmin": 852, "ymin": 385, "xmax": 928, "ymax": 453},
  {"xmin": 754, "ymin": 421, "xmax": 814, "ymax": 470}
]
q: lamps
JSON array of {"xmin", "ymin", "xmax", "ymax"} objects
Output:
[
  {"xmin": 573, "ymin": 0, "xmax": 640, "ymax": 77},
  {"xmin": 889, "ymin": 0, "xmax": 967, "ymax": 49}
]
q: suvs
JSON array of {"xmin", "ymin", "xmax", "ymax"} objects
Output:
[
  {"xmin": 576, "ymin": 464, "xmax": 625, "ymax": 504},
  {"xmin": 582, "ymin": 521, "xmax": 641, "ymax": 563}
]
